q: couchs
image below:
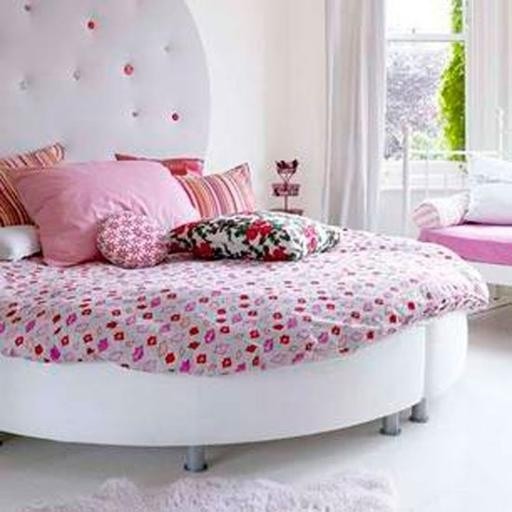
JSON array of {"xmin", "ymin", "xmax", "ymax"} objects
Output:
[{"xmin": 411, "ymin": 191, "xmax": 512, "ymax": 288}]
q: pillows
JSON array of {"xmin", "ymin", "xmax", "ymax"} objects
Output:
[
  {"xmin": 174, "ymin": 162, "xmax": 256, "ymax": 221},
  {"xmin": 8, "ymin": 160, "xmax": 202, "ymax": 267},
  {"xmin": 114, "ymin": 154, "xmax": 203, "ymax": 176},
  {"xmin": 0, "ymin": 142, "xmax": 65, "ymax": 226},
  {"xmin": 0, "ymin": 225, "xmax": 42, "ymax": 263},
  {"xmin": 463, "ymin": 157, "xmax": 512, "ymax": 225},
  {"xmin": 166, "ymin": 211, "xmax": 342, "ymax": 262},
  {"xmin": 95, "ymin": 212, "xmax": 167, "ymax": 269}
]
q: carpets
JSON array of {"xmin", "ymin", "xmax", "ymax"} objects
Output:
[{"xmin": 12, "ymin": 475, "xmax": 394, "ymax": 512}]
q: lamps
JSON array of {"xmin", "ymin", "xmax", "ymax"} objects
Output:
[{"xmin": 270, "ymin": 158, "xmax": 304, "ymax": 215}]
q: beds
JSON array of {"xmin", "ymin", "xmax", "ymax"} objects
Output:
[{"xmin": 0, "ymin": 0, "xmax": 490, "ymax": 472}]
